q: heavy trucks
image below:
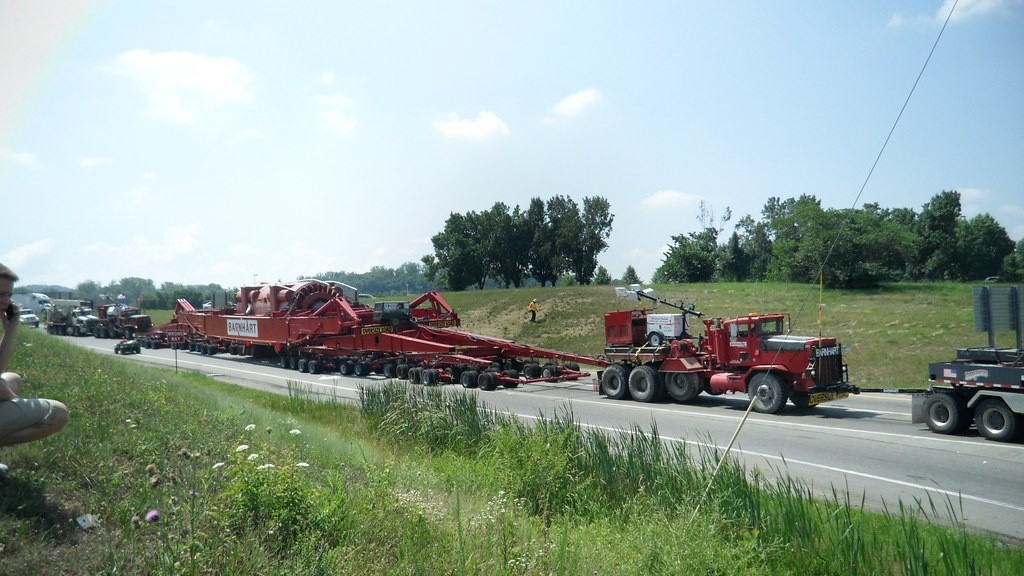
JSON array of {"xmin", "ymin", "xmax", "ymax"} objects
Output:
[{"xmin": 593, "ymin": 284, "xmax": 853, "ymax": 412}]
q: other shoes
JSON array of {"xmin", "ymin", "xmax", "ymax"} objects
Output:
[{"xmin": 0, "ymin": 462, "xmax": 8, "ymax": 473}]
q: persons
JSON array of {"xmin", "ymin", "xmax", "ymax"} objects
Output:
[
  {"xmin": 0, "ymin": 263, "xmax": 68, "ymax": 474},
  {"xmin": 752, "ymin": 323, "xmax": 763, "ymax": 336},
  {"xmin": 120, "ymin": 336, "xmax": 127, "ymax": 342},
  {"xmin": 529, "ymin": 299, "xmax": 538, "ymax": 322}
]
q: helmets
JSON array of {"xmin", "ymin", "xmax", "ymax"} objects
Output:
[{"xmin": 533, "ymin": 299, "xmax": 536, "ymax": 302}]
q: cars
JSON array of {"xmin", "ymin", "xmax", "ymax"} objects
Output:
[
  {"xmin": 17, "ymin": 309, "xmax": 40, "ymax": 328},
  {"xmin": 203, "ymin": 300, "xmax": 233, "ymax": 309}
]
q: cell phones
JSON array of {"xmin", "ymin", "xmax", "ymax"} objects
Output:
[{"xmin": 6, "ymin": 304, "xmax": 15, "ymax": 321}]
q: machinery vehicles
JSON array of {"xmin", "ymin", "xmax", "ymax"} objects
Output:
[
  {"xmin": 95, "ymin": 295, "xmax": 154, "ymax": 342},
  {"xmin": 46, "ymin": 298, "xmax": 100, "ymax": 338}
]
